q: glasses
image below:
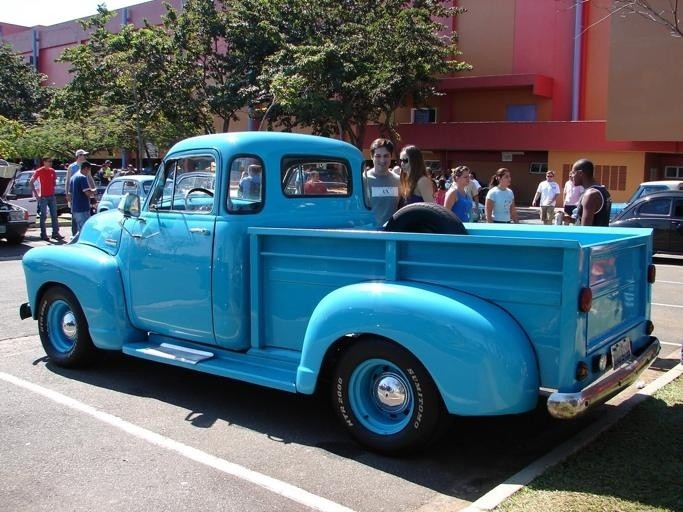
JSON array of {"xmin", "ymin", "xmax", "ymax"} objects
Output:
[{"xmin": 398, "ymin": 157, "xmax": 410, "ymax": 163}]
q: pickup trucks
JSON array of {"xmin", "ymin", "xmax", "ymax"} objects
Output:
[{"xmin": 13, "ymin": 133, "xmax": 661, "ymax": 452}]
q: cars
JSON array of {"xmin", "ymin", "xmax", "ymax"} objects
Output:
[{"xmin": 604, "ymin": 178, "xmax": 682, "ymax": 258}]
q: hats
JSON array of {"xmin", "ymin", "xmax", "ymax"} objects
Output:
[
  {"xmin": 80, "ymin": 160, "xmax": 90, "ymax": 170},
  {"xmin": 75, "ymin": 149, "xmax": 90, "ymax": 157},
  {"xmin": 127, "ymin": 164, "xmax": 134, "ymax": 168},
  {"xmin": 105, "ymin": 160, "xmax": 112, "ymax": 164}
]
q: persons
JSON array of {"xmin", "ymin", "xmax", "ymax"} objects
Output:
[
  {"xmin": 29, "ymin": 155, "xmax": 64, "ymax": 240},
  {"xmin": 398, "ymin": 144, "xmax": 433, "ymax": 208},
  {"xmin": 361, "ymin": 138, "xmax": 401, "ymax": 223}
]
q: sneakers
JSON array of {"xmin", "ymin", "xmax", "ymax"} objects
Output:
[{"xmin": 40, "ymin": 234, "xmax": 65, "ymax": 241}]
